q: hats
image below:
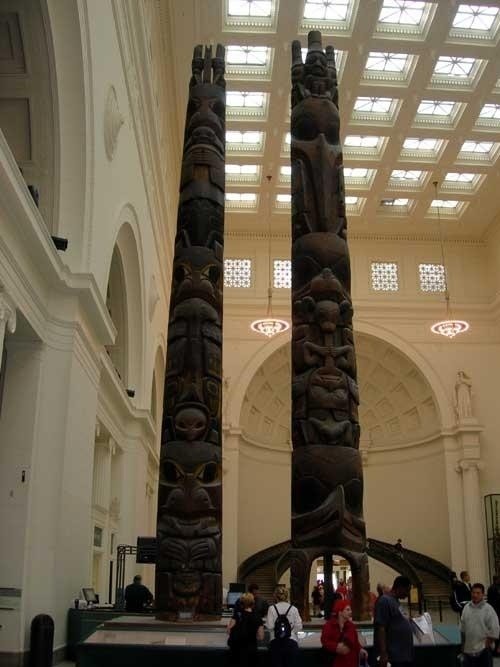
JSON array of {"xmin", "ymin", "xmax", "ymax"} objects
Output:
[{"xmin": 332, "ymin": 599, "xmax": 351, "ymax": 612}]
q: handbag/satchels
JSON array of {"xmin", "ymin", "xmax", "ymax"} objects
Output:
[{"xmin": 412, "ymin": 613, "xmax": 435, "ymax": 646}]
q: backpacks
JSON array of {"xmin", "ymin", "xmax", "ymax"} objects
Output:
[
  {"xmin": 449, "ymin": 582, "xmax": 463, "ymax": 611},
  {"xmin": 270, "ymin": 601, "xmax": 294, "ymax": 641}
]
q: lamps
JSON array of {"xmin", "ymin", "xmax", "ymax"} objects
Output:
[
  {"xmin": 250, "ymin": 175, "xmax": 290, "ymax": 339},
  {"xmin": 430, "ymin": 181, "xmax": 470, "ymax": 340}
]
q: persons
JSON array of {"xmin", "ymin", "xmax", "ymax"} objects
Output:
[
  {"xmin": 372, "ymin": 573, "xmax": 414, "ymax": 667},
  {"xmin": 393, "ymin": 538, "xmax": 403, "ymax": 555},
  {"xmin": 232, "ymin": 582, "xmax": 267, "ymax": 619},
  {"xmin": 312, "ymin": 585, "xmax": 322, "ymax": 617},
  {"xmin": 265, "ymin": 586, "xmax": 303, "ymax": 667},
  {"xmin": 320, "ymin": 599, "xmax": 368, "ymax": 666},
  {"xmin": 124, "ymin": 574, "xmax": 154, "ymax": 611},
  {"xmin": 337, "ymin": 579, "xmax": 346, "ymax": 600},
  {"xmin": 487, "ymin": 574, "xmax": 500, "ymax": 657},
  {"xmin": 457, "ymin": 570, "xmax": 473, "ymax": 615},
  {"xmin": 457, "ymin": 582, "xmax": 499, "ymax": 666},
  {"xmin": 227, "ymin": 592, "xmax": 264, "ymax": 666}
]
